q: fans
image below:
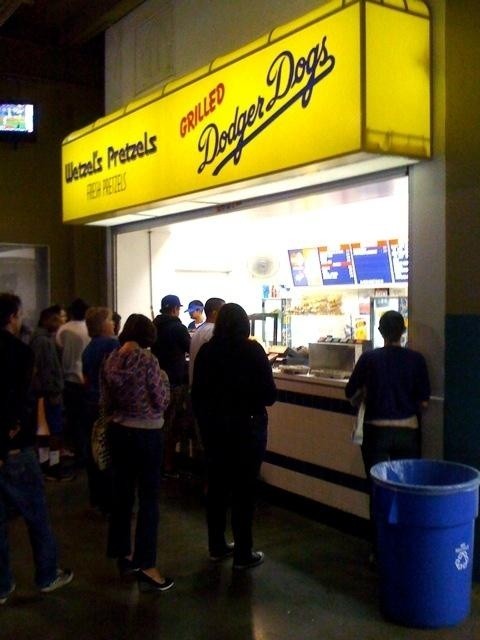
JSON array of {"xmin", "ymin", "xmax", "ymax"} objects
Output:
[{"xmin": 246, "ymin": 251, "xmax": 278, "ymax": 298}]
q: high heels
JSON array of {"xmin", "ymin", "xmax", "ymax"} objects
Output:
[
  {"xmin": 119, "ymin": 559, "xmax": 143, "ymax": 578},
  {"xmin": 137, "ymin": 569, "xmax": 176, "ymax": 593}
]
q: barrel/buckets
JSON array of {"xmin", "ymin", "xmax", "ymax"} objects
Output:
[{"xmin": 369, "ymin": 458, "xmax": 480, "ymax": 630}]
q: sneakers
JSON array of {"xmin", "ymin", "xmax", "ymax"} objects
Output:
[
  {"xmin": 231, "ymin": 550, "xmax": 266, "ymax": 571},
  {"xmin": 36, "ymin": 567, "xmax": 75, "ymax": 593},
  {"xmin": 208, "ymin": 541, "xmax": 235, "ymax": 561},
  {"xmin": 0, "ymin": 578, "xmax": 18, "ymax": 606}
]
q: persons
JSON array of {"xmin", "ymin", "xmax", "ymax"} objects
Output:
[
  {"xmin": 1, "ymin": 291, "xmax": 76, "ymax": 606},
  {"xmin": 341, "ymin": 309, "xmax": 431, "ymax": 565},
  {"xmin": 17, "ymin": 293, "xmax": 279, "ymax": 592}
]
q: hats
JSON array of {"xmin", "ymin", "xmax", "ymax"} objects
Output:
[
  {"xmin": 184, "ymin": 304, "xmax": 204, "ymax": 314},
  {"xmin": 161, "ymin": 294, "xmax": 183, "ymax": 307}
]
